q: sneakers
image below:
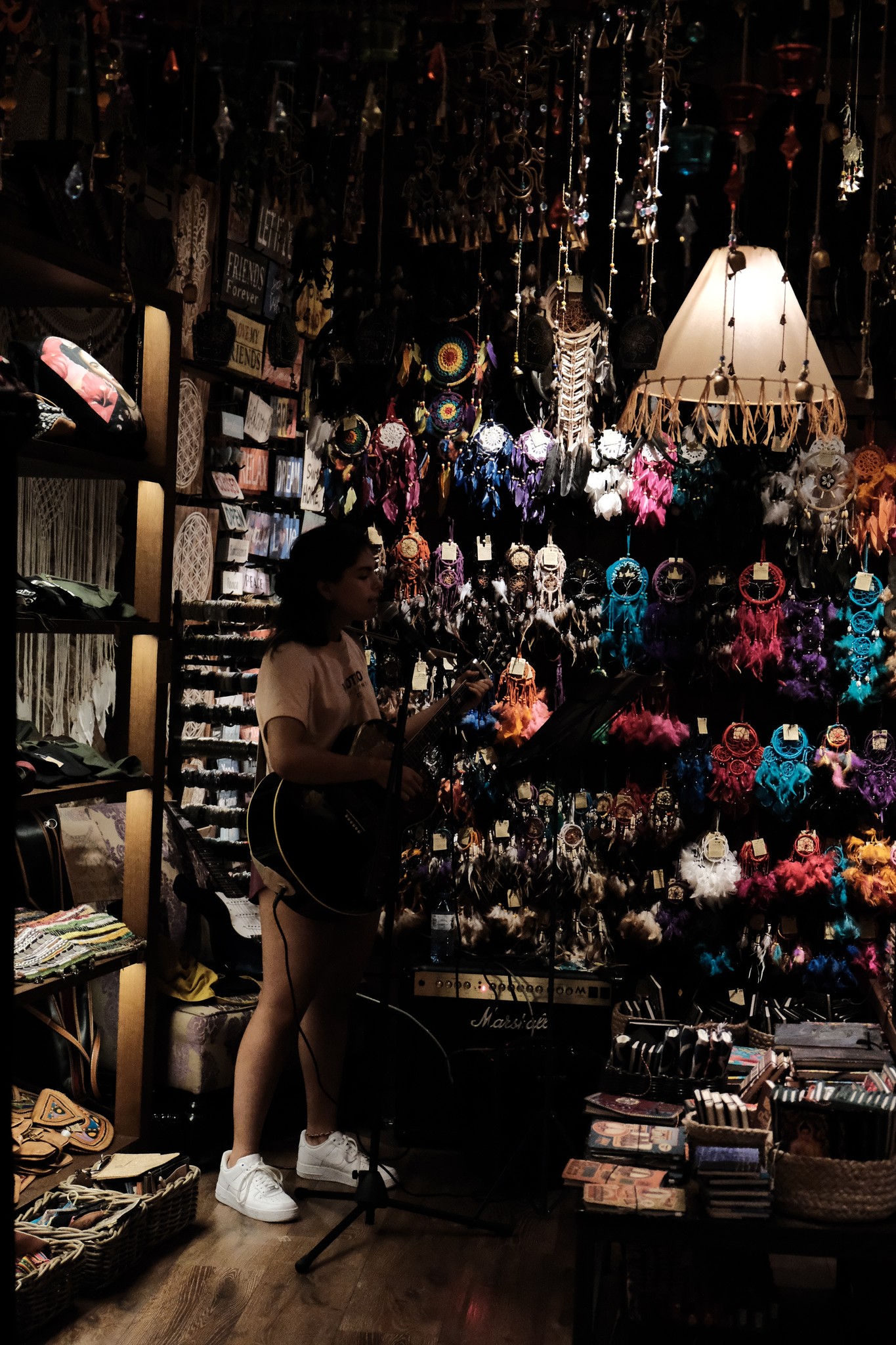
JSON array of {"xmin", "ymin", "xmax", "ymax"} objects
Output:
[
  {"xmin": 295, "ymin": 1128, "xmax": 398, "ymax": 1189},
  {"xmin": 215, "ymin": 1148, "xmax": 299, "ymax": 1223}
]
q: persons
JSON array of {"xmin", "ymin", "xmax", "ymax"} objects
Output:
[{"xmin": 214, "ymin": 522, "xmax": 494, "ymax": 1224}]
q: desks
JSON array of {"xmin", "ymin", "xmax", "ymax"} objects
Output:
[{"xmin": 580, "ymin": 940, "xmax": 896, "ymax": 1345}]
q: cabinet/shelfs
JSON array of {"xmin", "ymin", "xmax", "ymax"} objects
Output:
[{"xmin": 0, "ymin": 239, "xmax": 187, "ymax": 1214}]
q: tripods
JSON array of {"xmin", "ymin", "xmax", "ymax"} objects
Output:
[{"xmin": 294, "ymin": 622, "xmax": 512, "ymax": 1273}]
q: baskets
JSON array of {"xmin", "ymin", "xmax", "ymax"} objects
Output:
[{"xmin": 685, "ymin": 1096, "xmax": 896, "ymax": 1220}]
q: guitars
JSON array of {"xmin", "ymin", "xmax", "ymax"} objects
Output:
[{"xmin": 246, "ymin": 631, "xmax": 518, "ymax": 930}]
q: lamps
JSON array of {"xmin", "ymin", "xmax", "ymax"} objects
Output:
[{"xmin": 633, "ymin": 140, "xmax": 839, "ymax": 405}]
